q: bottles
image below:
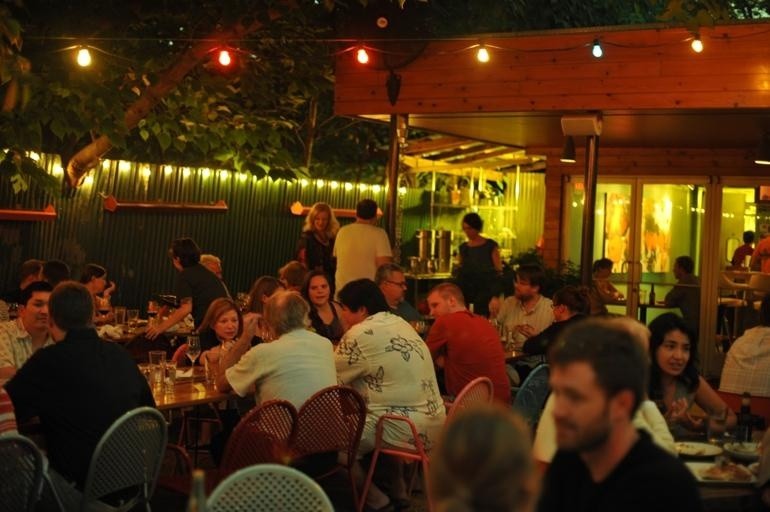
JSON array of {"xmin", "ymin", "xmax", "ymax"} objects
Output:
[
  {"xmin": 648, "ymin": 283, "xmax": 655, "ymax": 305},
  {"xmin": 737, "ymin": 391, "xmax": 752, "ymax": 442},
  {"xmin": 439, "ymin": 185, "xmax": 449, "ymax": 204},
  {"xmin": 451, "ymin": 183, "xmax": 461, "ymax": 205}
]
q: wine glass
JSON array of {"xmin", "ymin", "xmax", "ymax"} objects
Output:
[
  {"xmin": 184, "ymin": 334, "xmax": 201, "ymax": 375},
  {"xmin": 147, "ymin": 301, "xmax": 159, "ymax": 327},
  {"xmin": 94, "ymin": 299, "xmax": 109, "ymax": 327}
]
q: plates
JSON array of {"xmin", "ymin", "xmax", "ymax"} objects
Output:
[
  {"xmin": 674, "ymin": 441, "xmax": 723, "ymax": 459},
  {"xmin": 723, "ymin": 441, "xmax": 764, "ymax": 460},
  {"xmin": 684, "ymin": 461, "xmax": 760, "ymax": 485},
  {"xmin": 748, "ymin": 462, "xmax": 759, "ymax": 475}
]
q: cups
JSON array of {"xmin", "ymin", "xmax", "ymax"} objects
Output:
[
  {"xmin": 205, "ymin": 352, "xmax": 220, "ymax": 386},
  {"xmin": 638, "ymin": 289, "xmax": 647, "ymax": 305},
  {"xmin": 126, "ymin": 309, "xmax": 140, "ymax": 334},
  {"xmin": 163, "ymin": 359, "xmax": 177, "ymax": 393},
  {"xmin": 114, "ymin": 307, "xmax": 126, "ymax": 325},
  {"xmin": 707, "ymin": 424, "xmax": 725, "ymax": 445},
  {"xmin": 149, "ymin": 351, "xmax": 166, "ymax": 385}
]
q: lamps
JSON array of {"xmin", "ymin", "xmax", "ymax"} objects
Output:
[
  {"xmin": 559, "ymin": 135, "xmax": 578, "ymax": 163},
  {"xmin": 751, "ymin": 130, "xmax": 770, "ymax": 166}
]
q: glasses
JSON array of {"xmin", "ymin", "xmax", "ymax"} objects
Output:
[
  {"xmin": 550, "ymin": 304, "xmax": 558, "ymax": 310},
  {"xmin": 385, "ymin": 279, "xmax": 407, "ymax": 289}
]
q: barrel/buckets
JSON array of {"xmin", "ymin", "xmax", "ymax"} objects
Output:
[{"xmin": 413, "ymin": 227, "xmax": 453, "ymax": 273}]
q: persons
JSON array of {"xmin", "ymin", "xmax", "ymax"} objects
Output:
[
  {"xmin": 459, "ymin": 212, "xmax": 502, "ymax": 281},
  {"xmin": 298, "ymin": 201, "xmax": 339, "ymax": 299},
  {"xmin": 1, "ymin": 227, "xmax": 768, "ymax": 512},
  {"xmin": 332, "ymin": 198, "xmax": 395, "ymax": 306}
]
